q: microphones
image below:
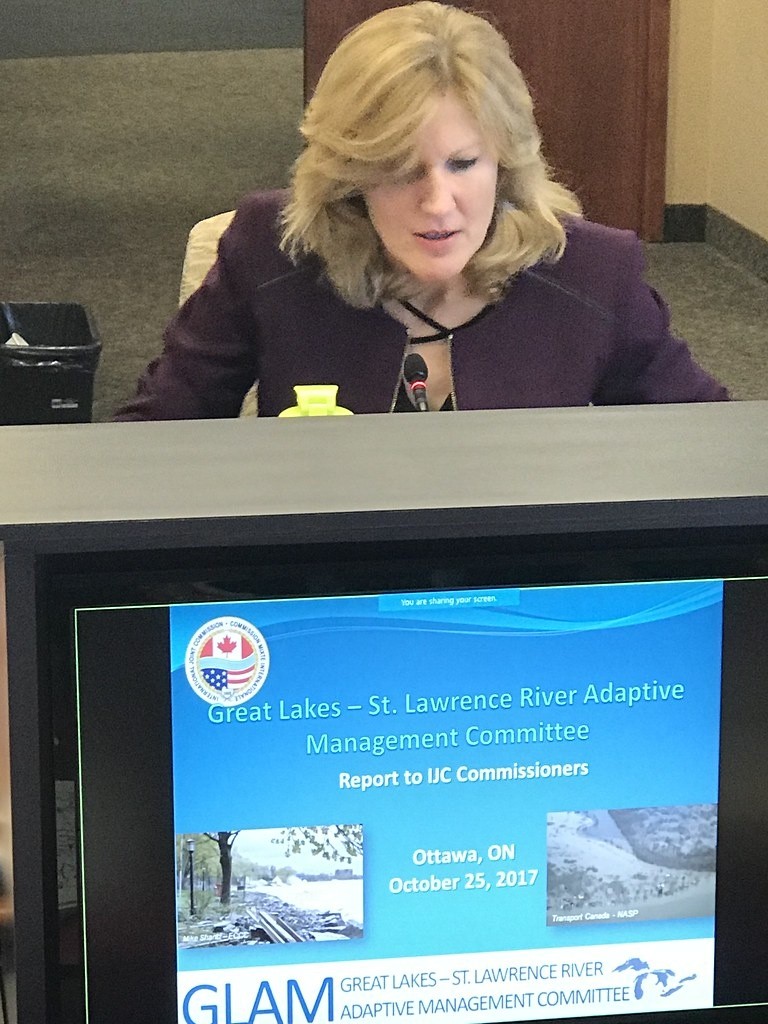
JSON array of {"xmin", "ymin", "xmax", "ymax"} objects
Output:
[{"xmin": 403, "ymin": 354, "xmax": 428, "ymax": 413}]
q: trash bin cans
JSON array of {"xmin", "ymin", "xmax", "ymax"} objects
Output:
[{"xmin": 0, "ymin": 298, "xmax": 105, "ymax": 428}]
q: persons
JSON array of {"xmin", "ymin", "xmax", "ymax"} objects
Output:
[{"xmin": 112, "ymin": 1, "xmax": 730, "ymax": 422}]
